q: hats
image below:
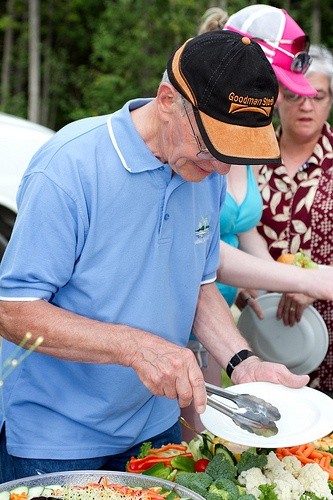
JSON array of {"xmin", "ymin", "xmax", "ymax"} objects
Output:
[
  {"xmin": 224, "ymin": 4, "xmax": 319, "ymax": 97},
  {"xmin": 167, "ymin": 30, "xmax": 282, "ymax": 165}
]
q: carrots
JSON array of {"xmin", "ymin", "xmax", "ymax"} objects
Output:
[{"xmin": 275, "ymin": 443, "xmax": 333, "ymax": 480}]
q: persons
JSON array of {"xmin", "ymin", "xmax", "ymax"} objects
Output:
[
  {"xmin": 198, "ymin": 4, "xmax": 333, "ymax": 310},
  {"xmin": 234, "ymin": 45, "xmax": 333, "ymax": 399},
  {"xmin": 0, "ymin": 30, "xmax": 281, "ymax": 485}
]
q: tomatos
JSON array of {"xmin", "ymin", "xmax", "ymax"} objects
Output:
[
  {"xmin": 194, "ymin": 459, "xmax": 210, "ymax": 472},
  {"xmin": 83, "ymin": 483, "xmax": 165, "ymax": 500}
]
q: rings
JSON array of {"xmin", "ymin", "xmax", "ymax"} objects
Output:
[
  {"xmin": 289, "ymin": 307, "xmax": 295, "ymax": 312},
  {"xmin": 245, "ymin": 297, "xmax": 251, "ymax": 303}
]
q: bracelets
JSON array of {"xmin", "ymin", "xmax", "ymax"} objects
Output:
[{"xmin": 226, "ymin": 349, "xmax": 257, "ymax": 379}]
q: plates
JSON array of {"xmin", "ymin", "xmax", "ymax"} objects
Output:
[
  {"xmin": 199, "ymin": 380, "xmax": 333, "ymax": 448},
  {"xmin": 236, "ymin": 293, "xmax": 329, "ymax": 375}
]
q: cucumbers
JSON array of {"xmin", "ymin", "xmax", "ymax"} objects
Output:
[{"xmin": 199, "ymin": 433, "xmax": 237, "ymax": 465}]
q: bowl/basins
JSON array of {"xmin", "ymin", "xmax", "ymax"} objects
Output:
[{"xmin": 0, "ymin": 470, "xmax": 207, "ymax": 500}]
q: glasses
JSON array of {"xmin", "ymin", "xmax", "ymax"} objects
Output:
[
  {"xmin": 266, "ymin": 41, "xmax": 313, "ymax": 75},
  {"xmin": 180, "ymin": 100, "xmax": 217, "ymax": 161}
]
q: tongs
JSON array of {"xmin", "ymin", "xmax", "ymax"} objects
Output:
[{"xmin": 205, "ymin": 382, "xmax": 281, "ymax": 437}]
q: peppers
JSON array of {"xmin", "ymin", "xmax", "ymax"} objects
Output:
[{"xmin": 127, "ymin": 444, "xmax": 197, "ymax": 483}]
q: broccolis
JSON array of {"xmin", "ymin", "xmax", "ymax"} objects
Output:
[{"xmin": 175, "ymin": 452, "xmax": 333, "ymax": 500}]
